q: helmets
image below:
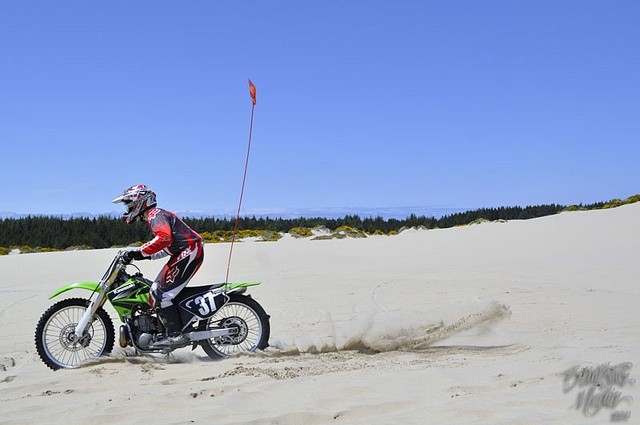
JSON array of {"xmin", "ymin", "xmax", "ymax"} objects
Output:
[{"xmin": 112, "ymin": 184, "xmax": 157, "ymax": 225}]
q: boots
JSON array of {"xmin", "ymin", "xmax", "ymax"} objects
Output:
[{"xmin": 152, "ymin": 305, "xmax": 184, "ymax": 345}]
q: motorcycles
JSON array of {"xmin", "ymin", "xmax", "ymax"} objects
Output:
[{"xmin": 34, "ymin": 250, "xmax": 270, "ymax": 373}]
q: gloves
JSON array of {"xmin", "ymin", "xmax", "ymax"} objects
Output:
[
  {"xmin": 134, "ymin": 253, "xmax": 151, "ymax": 260},
  {"xmin": 122, "ymin": 250, "xmax": 141, "ymax": 265}
]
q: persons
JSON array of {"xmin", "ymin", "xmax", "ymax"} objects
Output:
[{"xmin": 112, "ymin": 184, "xmax": 204, "ymax": 348}]
